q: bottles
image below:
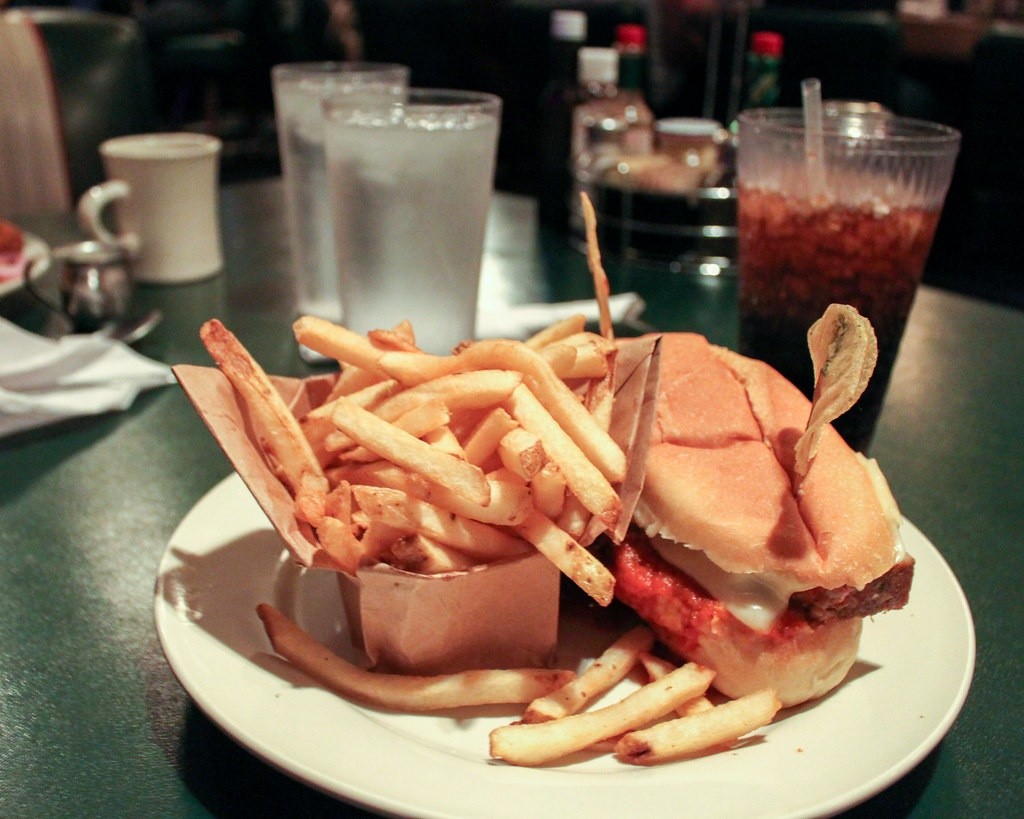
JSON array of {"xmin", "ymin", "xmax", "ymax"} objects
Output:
[
  {"xmin": 529, "ymin": 11, "xmax": 651, "ymax": 251},
  {"xmin": 725, "ymin": 30, "xmax": 794, "ymax": 143}
]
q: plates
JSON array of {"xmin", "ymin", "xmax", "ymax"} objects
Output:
[
  {"xmin": 0, "ymin": 229, "xmax": 51, "ymax": 298},
  {"xmin": 153, "ymin": 466, "xmax": 977, "ymax": 819}
]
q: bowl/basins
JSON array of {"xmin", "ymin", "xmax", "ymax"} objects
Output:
[{"xmin": 567, "ymin": 170, "xmax": 737, "ymax": 346}]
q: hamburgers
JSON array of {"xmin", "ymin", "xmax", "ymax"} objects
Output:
[{"xmin": 598, "ymin": 331, "xmax": 915, "ymax": 712}]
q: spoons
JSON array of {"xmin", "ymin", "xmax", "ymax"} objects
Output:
[{"xmin": 116, "ymin": 308, "xmax": 163, "ymax": 347}]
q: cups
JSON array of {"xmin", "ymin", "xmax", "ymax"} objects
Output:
[
  {"xmin": 321, "ymin": 85, "xmax": 504, "ymax": 368},
  {"xmin": 26, "ymin": 239, "xmax": 136, "ymax": 338},
  {"xmin": 270, "ymin": 60, "xmax": 407, "ymax": 365},
  {"xmin": 735, "ymin": 108, "xmax": 961, "ymax": 454},
  {"xmin": 77, "ymin": 132, "xmax": 225, "ymax": 286},
  {"xmin": 652, "ymin": 116, "xmax": 721, "ymax": 186}
]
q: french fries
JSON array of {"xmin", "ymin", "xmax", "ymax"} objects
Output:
[{"xmin": 200, "ymin": 309, "xmax": 780, "ymax": 767}]
